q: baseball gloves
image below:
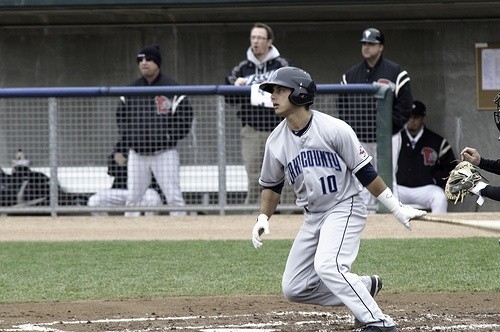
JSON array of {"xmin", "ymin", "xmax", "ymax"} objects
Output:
[{"xmin": 445, "ymin": 160, "xmax": 482, "ymax": 202}]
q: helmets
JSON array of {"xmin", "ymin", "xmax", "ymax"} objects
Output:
[
  {"xmin": 260, "ymin": 67, "xmax": 317, "ymax": 106},
  {"xmin": 493, "ymin": 91, "xmax": 500, "ymax": 134},
  {"xmin": 359, "ymin": 28, "xmax": 384, "ymax": 44}
]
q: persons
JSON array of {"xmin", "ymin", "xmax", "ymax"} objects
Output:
[
  {"xmin": 87, "ymin": 133, "xmax": 167, "ymax": 217},
  {"xmin": 336, "ymin": 27, "xmax": 414, "ymax": 215},
  {"xmin": 225, "ymin": 23, "xmax": 290, "ymax": 215},
  {"xmin": 396, "ymin": 101, "xmax": 458, "ymax": 213},
  {"xmin": 252, "ymin": 67, "xmax": 427, "ymax": 332},
  {"xmin": 117, "ymin": 46, "xmax": 193, "ymax": 216},
  {"xmin": 445, "ymin": 91, "xmax": 500, "ymax": 207}
]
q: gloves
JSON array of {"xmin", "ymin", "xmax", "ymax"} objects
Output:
[
  {"xmin": 391, "ymin": 202, "xmax": 427, "ymax": 232},
  {"xmin": 252, "ymin": 214, "xmax": 270, "ymax": 248}
]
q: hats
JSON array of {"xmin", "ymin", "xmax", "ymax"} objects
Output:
[
  {"xmin": 412, "ymin": 100, "xmax": 426, "ymax": 117},
  {"xmin": 137, "ymin": 46, "xmax": 161, "ymax": 61}
]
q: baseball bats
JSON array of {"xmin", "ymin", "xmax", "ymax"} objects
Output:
[{"xmin": 410, "ymin": 215, "xmax": 500, "ymax": 233}]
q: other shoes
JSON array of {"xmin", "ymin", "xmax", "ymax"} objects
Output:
[
  {"xmin": 364, "ymin": 326, "xmax": 401, "ymax": 332},
  {"xmin": 353, "ymin": 275, "xmax": 382, "ymax": 328}
]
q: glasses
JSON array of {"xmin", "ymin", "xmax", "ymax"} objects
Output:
[{"xmin": 137, "ymin": 57, "xmax": 154, "ymax": 62}]
